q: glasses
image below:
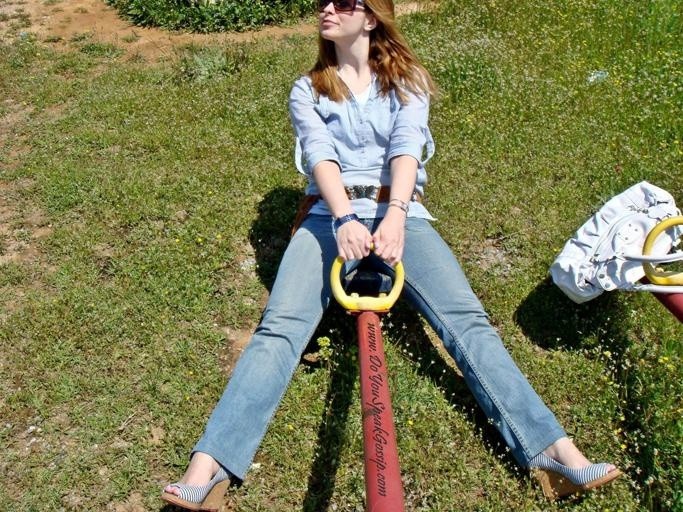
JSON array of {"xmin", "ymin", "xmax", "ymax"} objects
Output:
[{"xmin": 311, "ymin": 0, "xmax": 370, "ymax": 15}]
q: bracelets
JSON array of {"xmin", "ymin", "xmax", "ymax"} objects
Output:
[
  {"xmin": 387, "ymin": 199, "xmax": 409, "ymax": 213},
  {"xmin": 333, "ymin": 213, "xmax": 359, "ymax": 232}
]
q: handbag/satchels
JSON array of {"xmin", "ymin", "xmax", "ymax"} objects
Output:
[{"xmin": 548, "ymin": 180, "xmax": 683, "ymax": 322}]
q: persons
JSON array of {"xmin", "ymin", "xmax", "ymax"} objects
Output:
[{"xmin": 158, "ymin": 0, "xmax": 625, "ymax": 511}]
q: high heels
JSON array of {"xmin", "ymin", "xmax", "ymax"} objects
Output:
[
  {"xmin": 163, "ymin": 470, "xmax": 237, "ymax": 512},
  {"xmin": 527, "ymin": 448, "xmax": 622, "ymax": 498}
]
